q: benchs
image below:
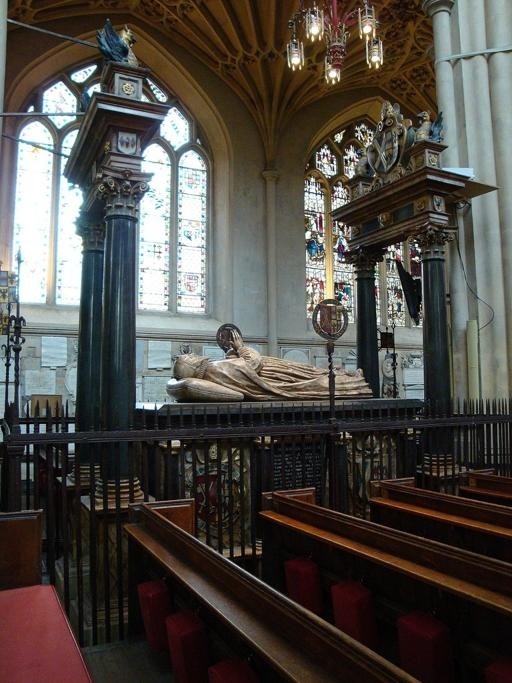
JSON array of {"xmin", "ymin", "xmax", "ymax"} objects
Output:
[
  {"xmin": 257, "ymin": 487, "xmax": 511, "ymax": 683},
  {"xmin": 459, "ymin": 468, "xmax": 512, "ymax": 506},
  {"xmin": 368, "ymin": 477, "xmax": 511, "ymax": 562},
  {"xmin": 121, "ymin": 496, "xmax": 416, "ymax": 681},
  {"xmin": 0, "ymin": 510, "xmax": 91, "ymax": 682}
]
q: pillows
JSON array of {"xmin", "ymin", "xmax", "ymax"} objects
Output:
[{"xmin": 166, "ymin": 379, "xmax": 245, "ymax": 402}]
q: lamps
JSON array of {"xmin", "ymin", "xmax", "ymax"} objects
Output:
[{"xmin": 285, "ymin": 0, "xmax": 383, "ymax": 85}]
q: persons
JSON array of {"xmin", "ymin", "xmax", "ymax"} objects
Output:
[{"xmin": 172, "ymin": 328, "xmax": 375, "ymax": 400}]
